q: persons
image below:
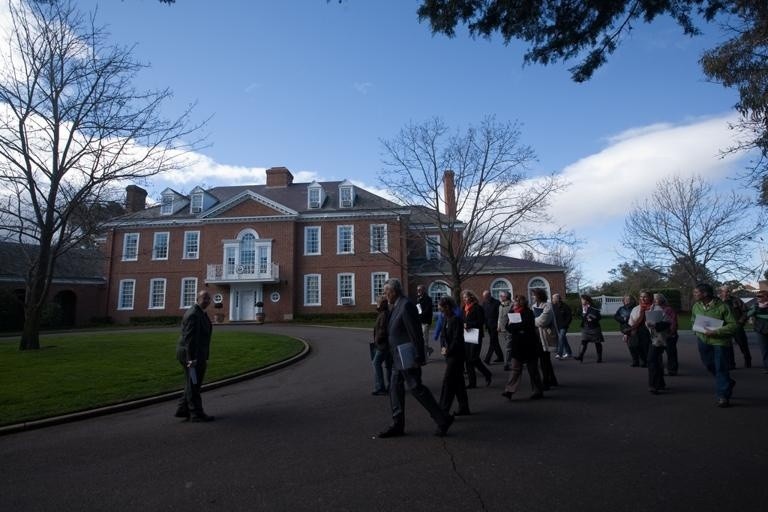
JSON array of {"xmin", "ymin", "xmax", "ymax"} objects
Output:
[
  {"xmin": 371, "ymin": 277, "xmax": 604, "ymax": 438},
  {"xmin": 175, "ymin": 291, "xmax": 215, "ymax": 423},
  {"xmin": 614, "ymin": 284, "xmax": 767, "ymax": 408}
]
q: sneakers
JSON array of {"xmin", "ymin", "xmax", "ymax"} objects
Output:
[
  {"xmin": 378, "ymin": 426, "xmax": 394, "ymax": 438},
  {"xmin": 434, "ymin": 416, "xmax": 455, "ymax": 437}
]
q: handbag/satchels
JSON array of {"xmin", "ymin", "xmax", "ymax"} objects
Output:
[{"xmin": 545, "ymin": 327, "xmax": 559, "ymax": 347}]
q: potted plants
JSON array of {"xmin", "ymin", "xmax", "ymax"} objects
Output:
[
  {"xmin": 253, "ymin": 301, "xmax": 266, "ymax": 322},
  {"xmin": 214, "ymin": 303, "xmax": 226, "ymax": 324}
]
